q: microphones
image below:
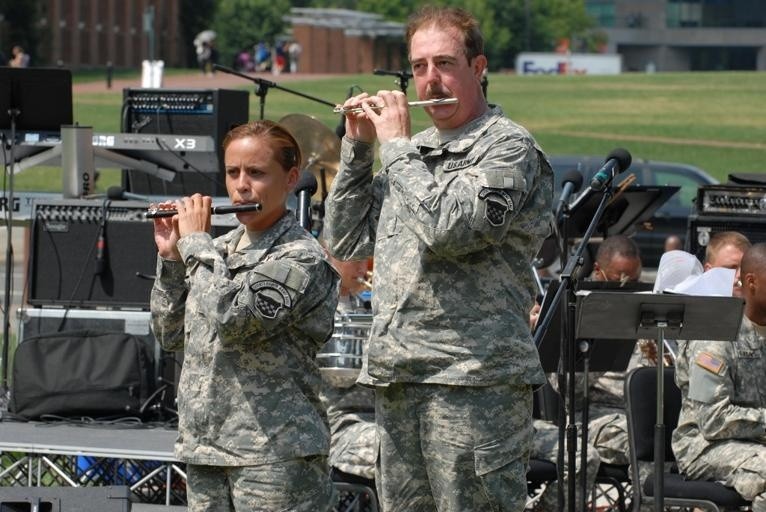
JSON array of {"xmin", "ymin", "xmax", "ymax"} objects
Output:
[
  {"xmin": 107, "ymin": 186, "xmax": 149, "ymax": 200},
  {"xmin": 554, "ymin": 170, "xmax": 583, "ymax": 224},
  {"xmin": 294, "ymin": 171, "xmax": 317, "ymax": 234},
  {"xmin": 587, "ymin": 148, "xmax": 631, "ymax": 190}
]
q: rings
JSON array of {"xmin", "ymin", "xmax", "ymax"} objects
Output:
[{"xmin": 377, "ymin": 101, "xmax": 388, "ymax": 111}]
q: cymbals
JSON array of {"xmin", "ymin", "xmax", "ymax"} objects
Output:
[{"xmin": 276, "ymin": 112, "xmax": 341, "ymax": 178}]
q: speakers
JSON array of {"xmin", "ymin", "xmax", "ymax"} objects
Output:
[
  {"xmin": 122, "ymin": 88, "xmax": 249, "ymax": 197},
  {"xmin": 26, "ymin": 198, "xmax": 159, "ymax": 309}
]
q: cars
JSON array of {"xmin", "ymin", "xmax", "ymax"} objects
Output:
[{"xmin": 550, "ymin": 155, "xmax": 720, "ymax": 269}]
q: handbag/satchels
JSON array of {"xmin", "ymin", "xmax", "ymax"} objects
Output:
[{"xmin": 9, "ymin": 330, "xmax": 154, "ymax": 421}]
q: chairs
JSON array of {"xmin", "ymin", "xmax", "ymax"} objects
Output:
[{"xmin": 1, "ymin": 357, "xmax": 766, "ymax": 511}]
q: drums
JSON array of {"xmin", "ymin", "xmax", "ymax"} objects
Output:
[{"xmin": 315, "ymin": 321, "xmax": 373, "ymax": 377}]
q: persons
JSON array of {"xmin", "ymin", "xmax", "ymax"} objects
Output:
[
  {"xmin": 320, "ymin": 1, "xmax": 555, "ymax": 512},
  {"xmin": 564, "ymin": 230, "xmax": 765, "ymax": 512},
  {"xmin": 317, "ymin": 227, "xmax": 601, "ymax": 512},
  {"xmin": 194, "ymin": 28, "xmax": 301, "ymax": 77},
  {"xmin": 148, "ymin": 119, "xmax": 343, "ymax": 512},
  {"xmin": 6, "ymin": 45, "xmax": 30, "ymax": 68}
]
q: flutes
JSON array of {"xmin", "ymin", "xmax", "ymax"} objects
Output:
[
  {"xmin": 332, "ymin": 97, "xmax": 459, "ymax": 114},
  {"xmin": 143, "ymin": 203, "xmax": 263, "ymax": 218}
]
